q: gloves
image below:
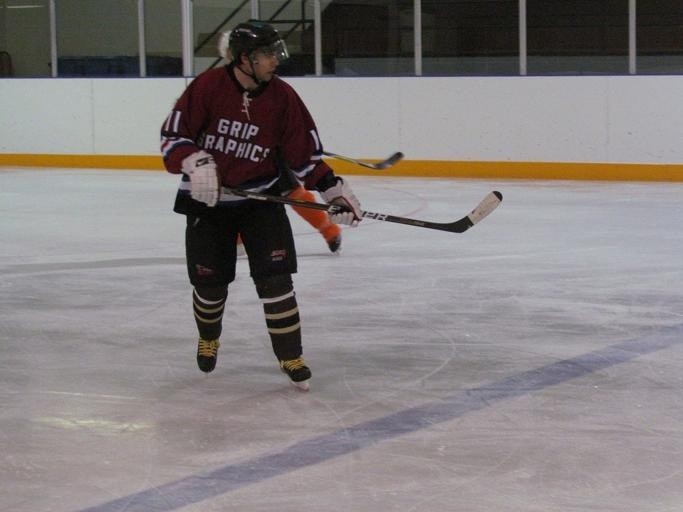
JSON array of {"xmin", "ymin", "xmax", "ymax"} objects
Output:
[
  {"xmin": 181, "ymin": 149, "xmax": 222, "ymax": 208},
  {"xmin": 320, "ymin": 177, "xmax": 363, "ymax": 227}
]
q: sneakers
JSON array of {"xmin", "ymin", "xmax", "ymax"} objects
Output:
[
  {"xmin": 280, "ymin": 357, "xmax": 312, "ymax": 381},
  {"xmin": 197, "ymin": 336, "xmax": 220, "ymax": 373},
  {"xmin": 329, "ymin": 234, "xmax": 341, "ymax": 252}
]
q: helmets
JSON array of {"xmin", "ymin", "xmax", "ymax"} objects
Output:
[{"xmin": 218, "ymin": 19, "xmax": 290, "ymax": 60}]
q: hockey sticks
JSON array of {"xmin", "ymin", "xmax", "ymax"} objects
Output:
[
  {"xmin": 176, "ymin": 180, "xmax": 503, "ymax": 232},
  {"xmin": 319, "ymin": 151, "xmax": 404, "ymax": 170}
]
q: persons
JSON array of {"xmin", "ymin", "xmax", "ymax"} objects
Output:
[
  {"xmin": 216, "ymin": 30, "xmax": 342, "ymax": 261},
  {"xmin": 160, "ymin": 18, "xmax": 365, "ymax": 383}
]
